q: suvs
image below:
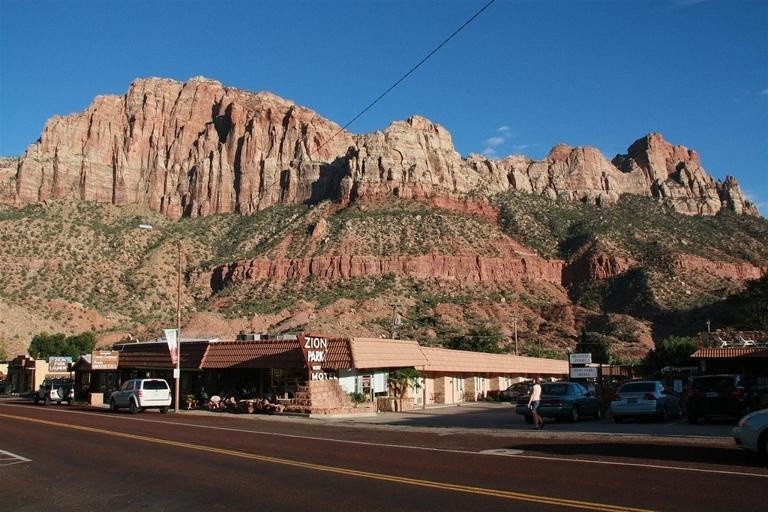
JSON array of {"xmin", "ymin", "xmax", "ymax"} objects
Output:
[
  {"xmin": 33, "ymin": 378, "xmax": 75, "ymax": 406},
  {"xmin": 110, "ymin": 379, "xmax": 173, "ymax": 414}
]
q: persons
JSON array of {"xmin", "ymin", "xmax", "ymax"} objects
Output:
[{"xmin": 527, "ymin": 375, "xmax": 544, "ymax": 430}]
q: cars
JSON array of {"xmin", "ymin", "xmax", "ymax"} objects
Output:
[
  {"xmin": 516, "ymin": 381, "xmax": 602, "ymax": 424},
  {"xmin": 609, "ymin": 374, "xmax": 768, "ymax": 462},
  {"xmin": 500, "ymin": 382, "xmax": 536, "ymax": 402}
]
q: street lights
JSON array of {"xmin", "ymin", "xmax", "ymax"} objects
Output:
[{"xmin": 138, "ymin": 224, "xmax": 182, "ymax": 412}]
